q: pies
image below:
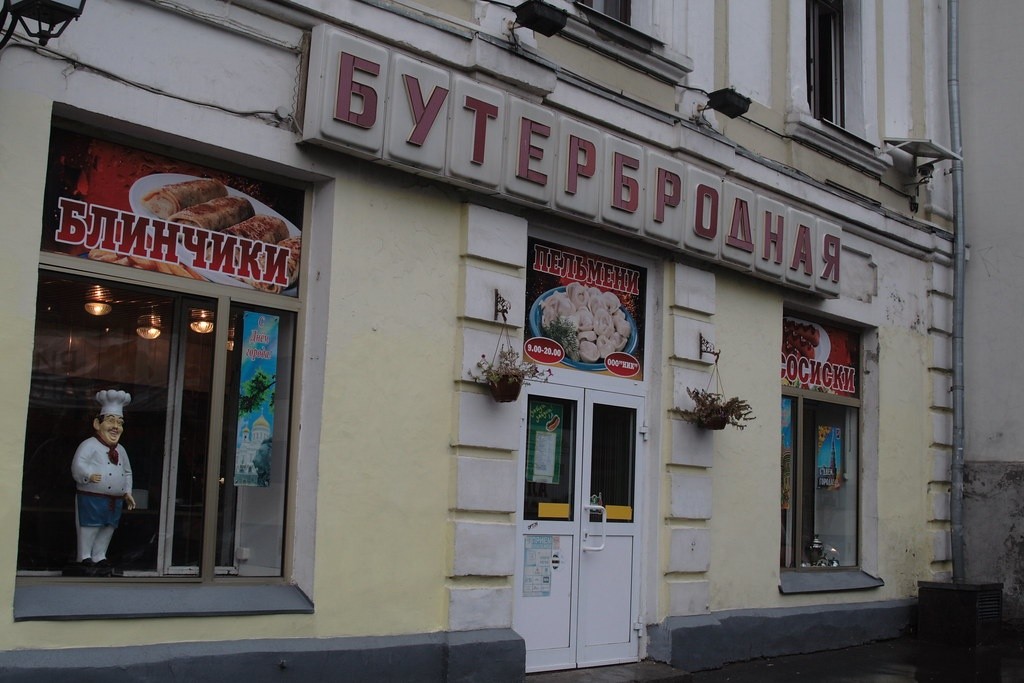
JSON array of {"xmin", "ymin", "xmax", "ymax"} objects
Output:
[{"xmin": 141, "ymin": 176, "xmax": 301, "ymax": 293}]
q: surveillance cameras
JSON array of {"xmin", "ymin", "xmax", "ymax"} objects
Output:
[{"xmin": 917, "ymin": 164, "xmax": 935, "ymax": 178}]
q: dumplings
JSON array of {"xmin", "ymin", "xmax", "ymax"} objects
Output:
[{"xmin": 541, "ymin": 282, "xmax": 631, "ymax": 364}]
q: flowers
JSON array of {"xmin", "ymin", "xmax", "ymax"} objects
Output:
[
  {"xmin": 668, "ymin": 386, "xmax": 757, "ymax": 431},
  {"xmin": 468, "ymin": 342, "xmax": 554, "ymax": 388}
]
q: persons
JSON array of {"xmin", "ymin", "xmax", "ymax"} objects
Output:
[{"xmin": 72, "ymin": 390, "xmax": 135, "ymax": 566}]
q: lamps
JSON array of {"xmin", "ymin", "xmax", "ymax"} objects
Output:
[
  {"xmin": 190, "ymin": 321, "xmax": 213, "ymax": 333},
  {"xmin": 84, "ymin": 302, "xmax": 112, "ymax": 316},
  {"xmin": 693, "ymin": 89, "xmax": 752, "ymax": 120},
  {"xmin": 136, "ymin": 327, "xmax": 161, "ymax": 340},
  {"xmin": 0, "ymin": 0, "xmax": 87, "ymax": 49},
  {"xmin": 227, "ymin": 341, "xmax": 234, "ymax": 351},
  {"xmin": 501, "ymin": 0, "xmax": 572, "ymax": 46}
]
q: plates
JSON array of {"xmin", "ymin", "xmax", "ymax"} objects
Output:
[
  {"xmin": 781, "ymin": 316, "xmax": 831, "ymax": 373},
  {"xmin": 529, "ymin": 285, "xmax": 639, "ymax": 372},
  {"xmin": 129, "ymin": 173, "xmax": 302, "ymax": 291}
]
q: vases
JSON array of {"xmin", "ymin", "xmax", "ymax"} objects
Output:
[
  {"xmin": 489, "ymin": 374, "xmax": 524, "ymax": 403},
  {"xmin": 704, "ymin": 413, "xmax": 726, "ymax": 430}
]
